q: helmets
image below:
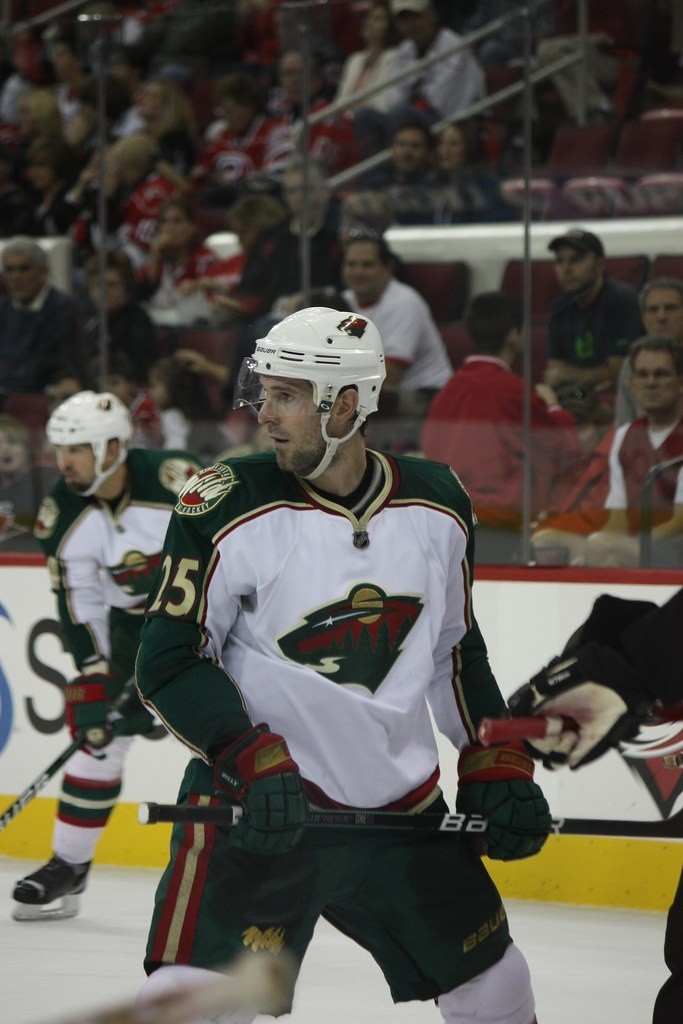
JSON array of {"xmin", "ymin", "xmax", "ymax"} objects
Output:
[
  {"xmin": 233, "ymin": 306, "xmax": 387, "ymax": 418},
  {"xmin": 45, "ymin": 391, "xmax": 134, "ymax": 463}
]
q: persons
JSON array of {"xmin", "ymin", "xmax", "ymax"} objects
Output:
[{"xmin": 0, "ymin": 1, "xmax": 683, "ymax": 1024}]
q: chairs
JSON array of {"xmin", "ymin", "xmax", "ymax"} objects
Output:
[{"xmin": 0, "ymin": 0, "xmax": 683, "ymax": 570}]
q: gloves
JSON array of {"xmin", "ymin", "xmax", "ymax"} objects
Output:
[
  {"xmin": 455, "ymin": 741, "xmax": 552, "ymax": 860},
  {"xmin": 214, "ymin": 722, "xmax": 310, "ymax": 857},
  {"xmin": 64, "ymin": 673, "xmax": 114, "ymax": 760},
  {"xmin": 507, "ymin": 642, "xmax": 651, "ymax": 770}
]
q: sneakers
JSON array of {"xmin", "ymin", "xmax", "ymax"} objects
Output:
[{"xmin": 12, "ymin": 855, "xmax": 91, "ymax": 921}]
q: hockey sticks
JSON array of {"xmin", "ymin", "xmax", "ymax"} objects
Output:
[
  {"xmin": 140, "ymin": 800, "xmax": 681, "ymax": 838},
  {"xmin": 1, "ymin": 736, "xmax": 86, "ymax": 827},
  {"xmin": 480, "ymin": 714, "xmax": 681, "ymax": 757}
]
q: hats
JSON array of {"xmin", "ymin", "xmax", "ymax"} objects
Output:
[
  {"xmin": 547, "ymin": 229, "xmax": 604, "ymax": 257},
  {"xmin": 390, "ymin": 0, "xmax": 431, "ymax": 17}
]
